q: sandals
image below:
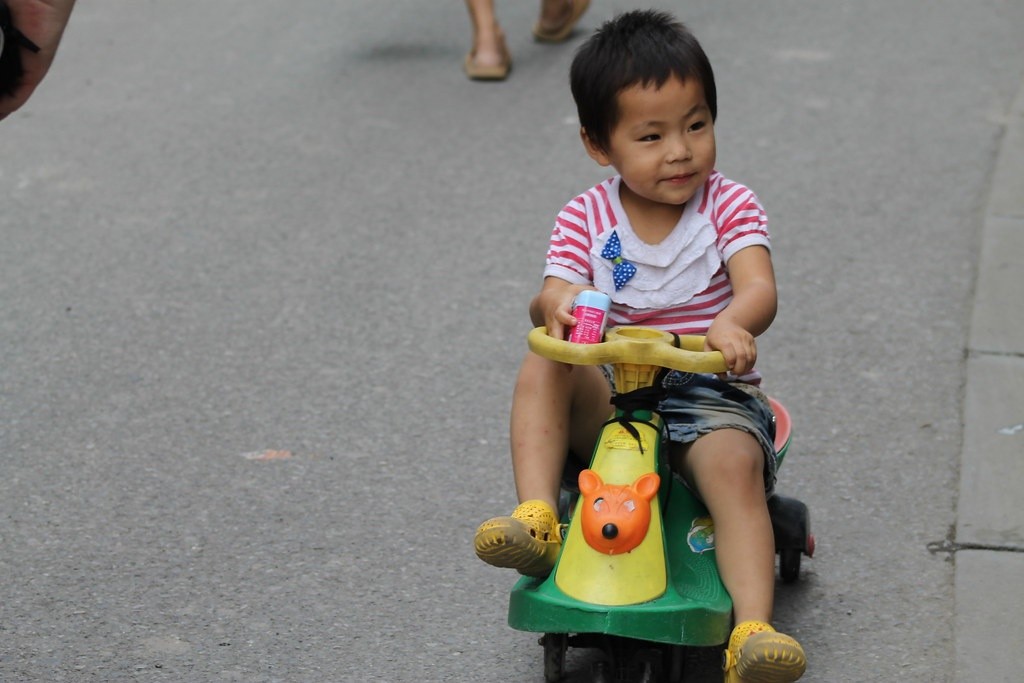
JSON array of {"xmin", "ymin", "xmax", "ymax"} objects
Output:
[
  {"xmin": 722, "ymin": 620, "xmax": 806, "ymax": 683},
  {"xmin": 474, "ymin": 499, "xmax": 567, "ymax": 577}
]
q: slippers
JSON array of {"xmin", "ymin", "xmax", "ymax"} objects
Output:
[
  {"xmin": 532, "ymin": 0, "xmax": 589, "ymax": 41},
  {"xmin": 463, "ymin": 46, "xmax": 511, "ymax": 79}
]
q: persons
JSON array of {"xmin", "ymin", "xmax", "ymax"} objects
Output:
[
  {"xmin": 0, "ymin": 0, "xmax": 76, "ymax": 125},
  {"xmin": 471, "ymin": 8, "xmax": 806, "ymax": 683},
  {"xmin": 464, "ymin": 0, "xmax": 590, "ymax": 82}
]
